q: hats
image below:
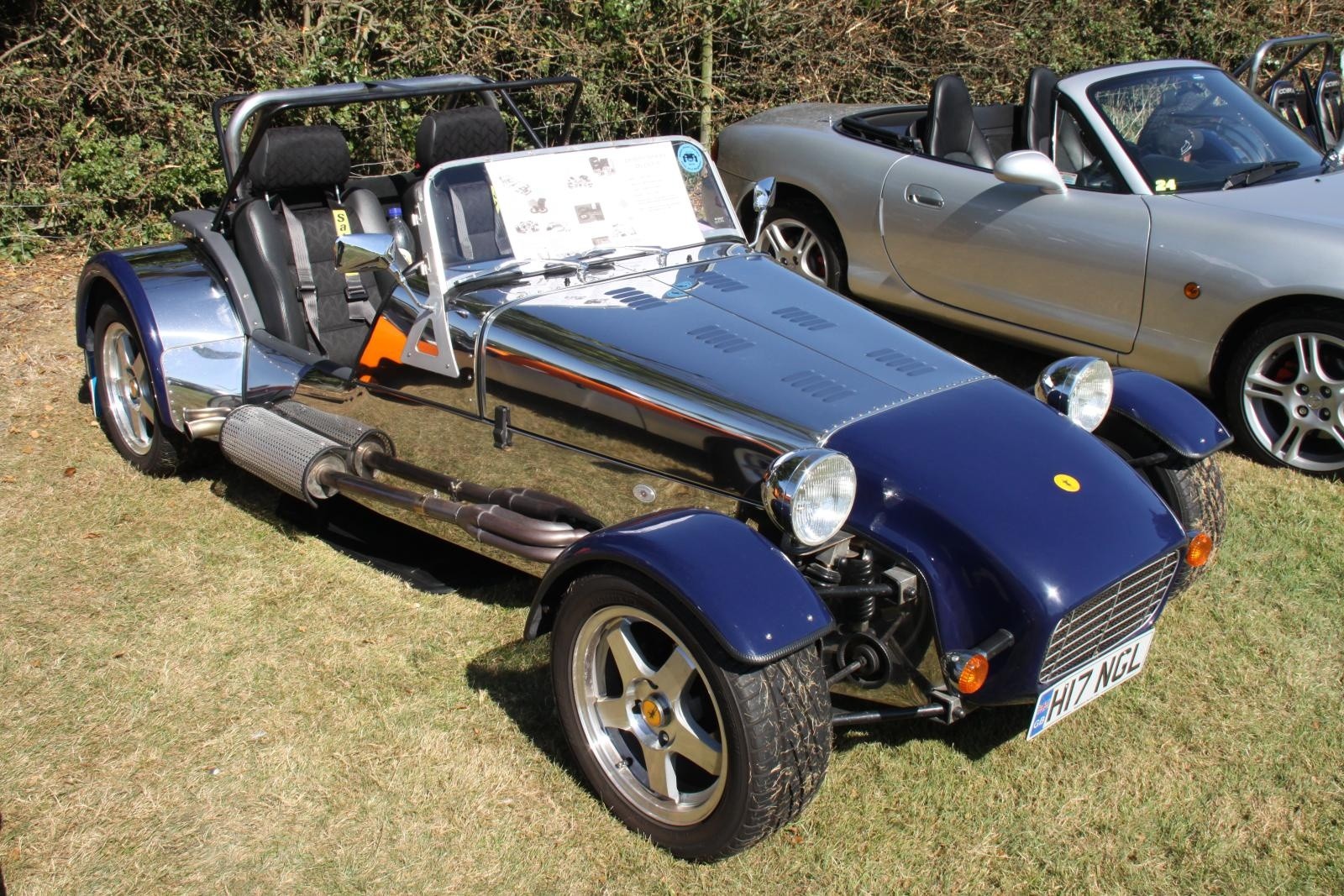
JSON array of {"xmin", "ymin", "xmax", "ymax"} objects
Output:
[{"xmin": 1148, "ymin": 124, "xmax": 1204, "ymax": 158}]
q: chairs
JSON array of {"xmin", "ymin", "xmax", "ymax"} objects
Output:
[
  {"xmin": 1270, "ymin": 80, "xmax": 1307, "ymax": 130},
  {"xmin": 1021, "ymin": 68, "xmax": 1093, "ymax": 173},
  {"xmin": 403, "ymin": 106, "xmax": 513, "ymax": 267},
  {"xmin": 238, "ymin": 125, "xmax": 390, "ymax": 351},
  {"xmin": 1314, "ymin": 71, "xmax": 1344, "ymax": 141},
  {"xmin": 923, "ymin": 75, "xmax": 996, "ymax": 170}
]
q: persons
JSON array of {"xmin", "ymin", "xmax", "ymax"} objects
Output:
[{"xmin": 1154, "ymin": 123, "xmax": 1242, "ymax": 164}]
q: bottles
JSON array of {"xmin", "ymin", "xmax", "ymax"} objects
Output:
[{"xmin": 383, "ymin": 207, "xmax": 414, "ymax": 274}]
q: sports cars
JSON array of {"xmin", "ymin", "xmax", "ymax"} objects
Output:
[
  {"xmin": 77, "ymin": 75, "xmax": 1239, "ymax": 858},
  {"xmin": 705, "ymin": 34, "xmax": 1343, "ymax": 480}
]
